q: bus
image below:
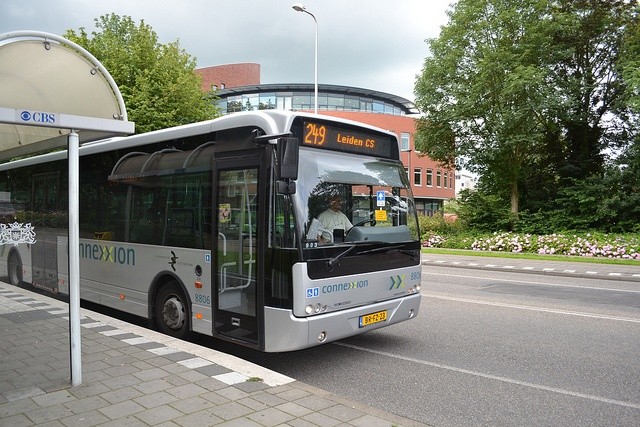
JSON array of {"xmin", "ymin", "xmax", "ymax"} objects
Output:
[{"xmin": 0, "ymin": 109, "xmax": 421, "ymax": 354}]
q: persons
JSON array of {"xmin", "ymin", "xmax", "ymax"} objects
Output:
[{"xmin": 306, "ymin": 192, "xmax": 354, "ymax": 242}]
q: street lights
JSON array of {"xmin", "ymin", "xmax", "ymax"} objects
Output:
[{"xmin": 292, "ymin": 3, "xmax": 318, "ymax": 113}]
